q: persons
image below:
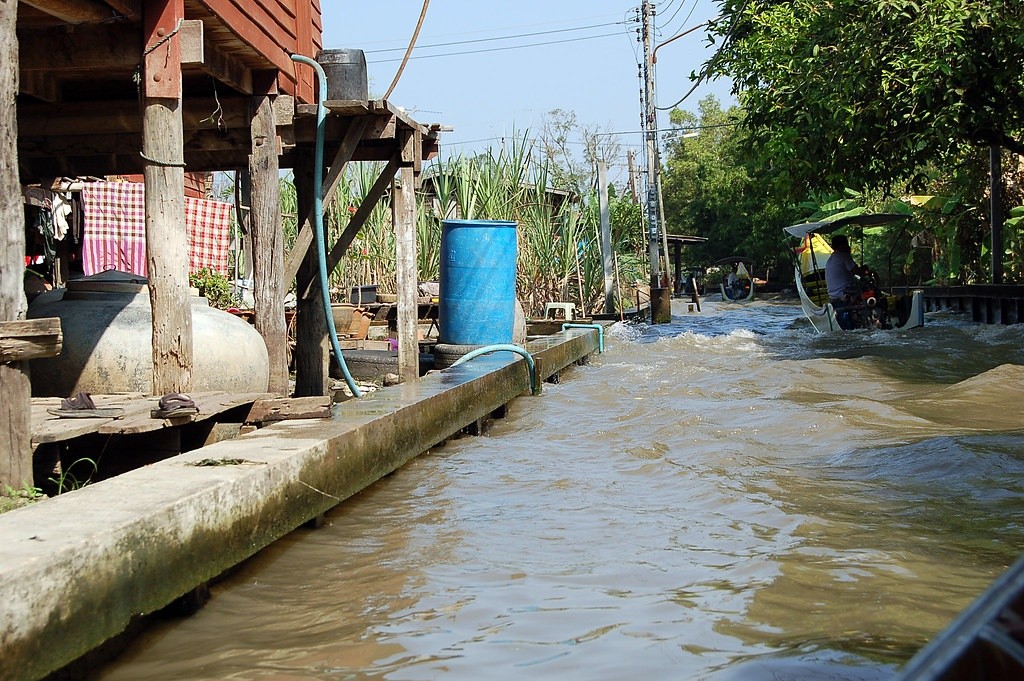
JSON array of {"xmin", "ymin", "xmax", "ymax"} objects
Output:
[
  {"xmin": 728, "ymin": 267, "xmax": 739, "ymax": 287},
  {"xmin": 825, "ymin": 234, "xmax": 893, "ymax": 330},
  {"xmin": 686, "ymin": 273, "xmax": 693, "ymax": 290}
]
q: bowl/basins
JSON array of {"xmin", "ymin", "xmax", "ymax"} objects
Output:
[{"xmin": 351, "ymin": 285, "xmax": 380, "ymax": 303}]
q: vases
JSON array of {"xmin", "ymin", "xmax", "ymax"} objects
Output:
[{"xmin": 329, "ymin": 285, "xmax": 380, "ymax": 343}]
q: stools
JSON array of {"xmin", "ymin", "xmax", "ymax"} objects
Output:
[{"xmin": 546, "ymin": 302, "xmax": 576, "ymax": 321}]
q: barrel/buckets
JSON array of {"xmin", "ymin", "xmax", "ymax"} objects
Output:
[
  {"xmin": 314, "ymin": 49, "xmax": 368, "ymax": 104},
  {"xmin": 438, "ymin": 219, "xmax": 518, "ymax": 345}
]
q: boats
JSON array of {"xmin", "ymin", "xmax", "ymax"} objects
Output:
[
  {"xmin": 715, "ymin": 256, "xmax": 756, "ymax": 302},
  {"xmin": 795, "ymin": 221, "xmax": 925, "ymax": 333},
  {"xmin": 679, "ymin": 267, "xmax": 707, "ymax": 296}
]
